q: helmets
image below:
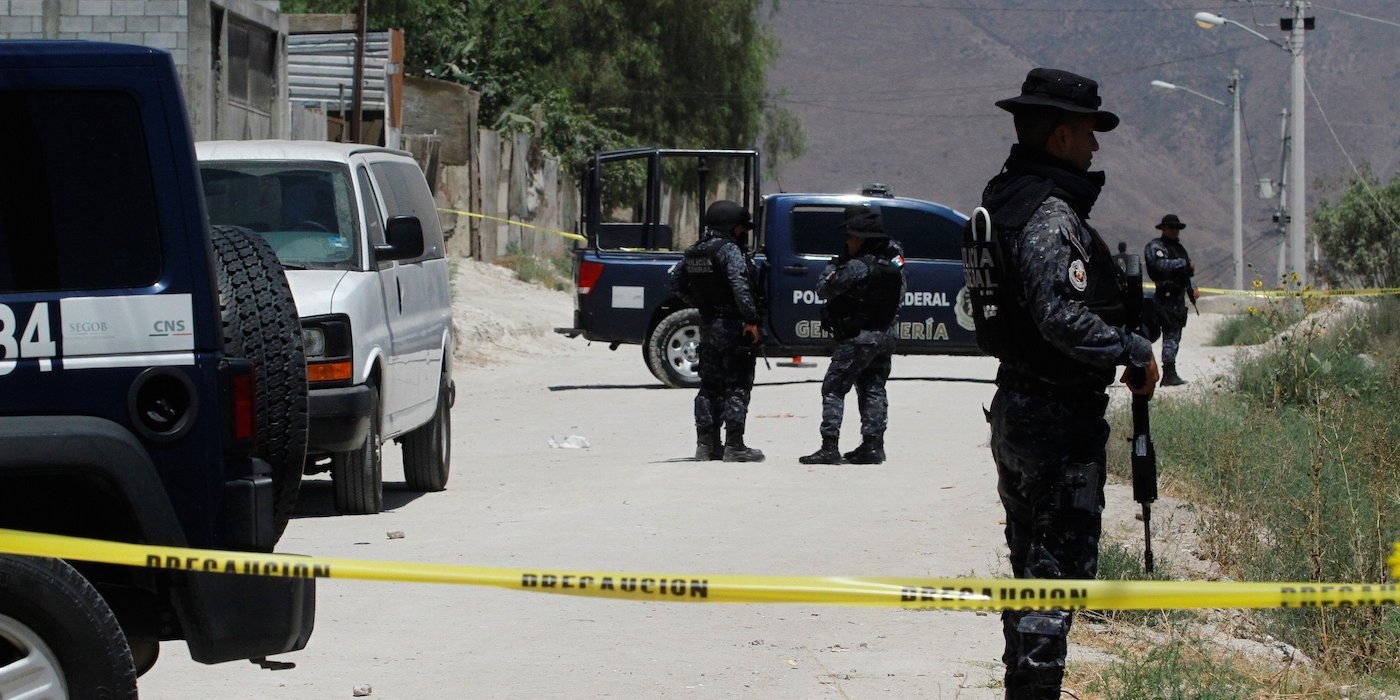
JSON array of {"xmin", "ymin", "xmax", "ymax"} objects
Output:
[{"xmin": 704, "ymin": 201, "xmax": 757, "ymax": 233}]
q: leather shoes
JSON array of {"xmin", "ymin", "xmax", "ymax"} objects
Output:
[
  {"xmin": 843, "ymin": 442, "xmax": 884, "ymax": 464},
  {"xmin": 799, "ymin": 448, "xmax": 840, "ymax": 464}
]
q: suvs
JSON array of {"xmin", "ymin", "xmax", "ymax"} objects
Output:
[{"xmin": 0, "ymin": 37, "xmax": 317, "ymax": 700}]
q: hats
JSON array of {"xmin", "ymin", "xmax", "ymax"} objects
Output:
[
  {"xmin": 994, "ymin": 68, "xmax": 1120, "ymax": 132},
  {"xmin": 1155, "ymin": 214, "xmax": 1187, "ymax": 230},
  {"xmin": 835, "ymin": 213, "xmax": 890, "ymax": 240}
]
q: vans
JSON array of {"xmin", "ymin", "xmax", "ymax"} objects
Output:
[{"xmin": 192, "ymin": 139, "xmax": 456, "ymax": 518}]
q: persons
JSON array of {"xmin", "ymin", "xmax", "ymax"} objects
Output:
[
  {"xmin": 1111, "ymin": 242, "xmax": 1128, "ymax": 274},
  {"xmin": 798, "ymin": 205, "xmax": 907, "ymax": 464},
  {"xmin": 666, "ymin": 200, "xmax": 765, "ymax": 463},
  {"xmin": 961, "ymin": 68, "xmax": 1160, "ymax": 700},
  {"xmin": 1145, "ymin": 214, "xmax": 1199, "ymax": 387}
]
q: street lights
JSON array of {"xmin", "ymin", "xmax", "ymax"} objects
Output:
[
  {"xmin": 1151, "ymin": 80, "xmax": 1243, "ymax": 290},
  {"xmin": 1193, "ymin": 12, "xmax": 1307, "ymax": 290}
]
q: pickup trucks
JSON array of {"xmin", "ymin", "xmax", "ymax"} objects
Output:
[{"xmin": 554, "ymin": 183, "xmax": 990, "ymax": 388}]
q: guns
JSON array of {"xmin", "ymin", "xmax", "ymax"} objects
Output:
[
  {"xmin": 734, "ymin": 259, "xmax": 771, "ymax": 358},
  {"xmin": 1116, "ymin": 252, "xmax": 1156, "ymax": 577},
  {"xmin": 1160, "ymin": 235, "xmax": 1198, "ymax": 314}
]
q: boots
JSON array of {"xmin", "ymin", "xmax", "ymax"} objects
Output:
[
  {"xmin": 723, "ymin": 432, "xmax": 765, "ymax": 462},
  {"xmin": 695, "ymin": 434, "xmax": 727, "ymax": 461},
  {"xmin": 1159, "ymin": 364, "xmax": 1188, "ymax": 387}
]
